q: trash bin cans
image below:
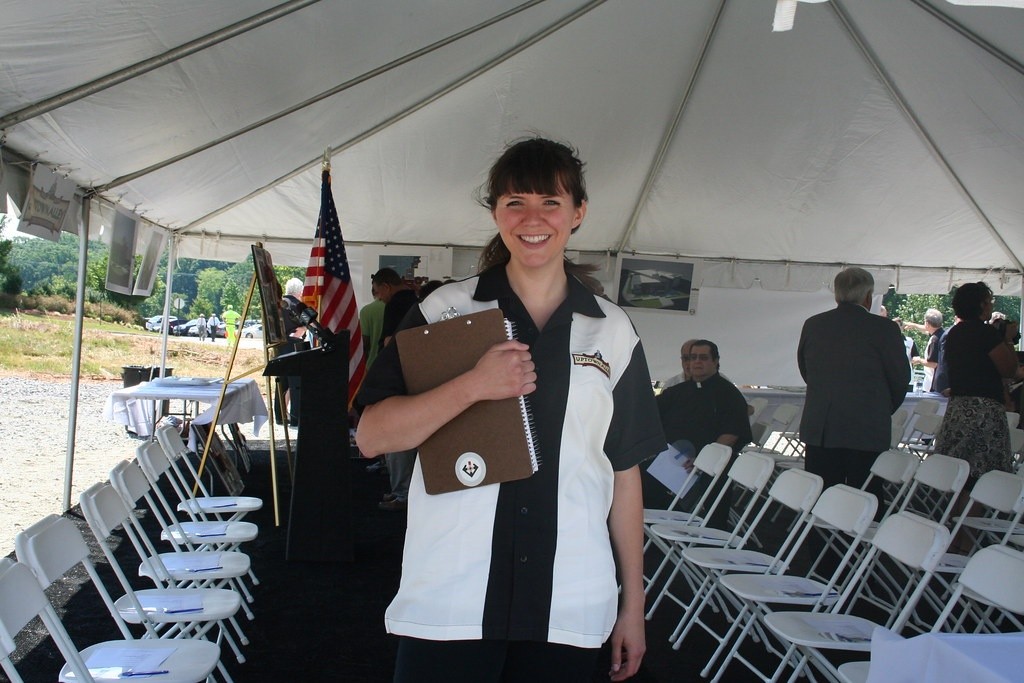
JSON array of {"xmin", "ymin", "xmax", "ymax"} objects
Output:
[{"xmin": 121, "ymin": 365, "xmax": 174, "ymax": 442}]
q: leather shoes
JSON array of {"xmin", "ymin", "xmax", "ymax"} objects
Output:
[
  {"xmin": 379, "ymin": 498, "xmax": 407, "ymax": 512},
  {"xmin": 383, "ymin": 493, "xmax": 397, "ymax": 502}
]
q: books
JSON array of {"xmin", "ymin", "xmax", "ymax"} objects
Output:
[{"xmin": 395, "ymin": 308, "xmax": 541, "ymax": 495}]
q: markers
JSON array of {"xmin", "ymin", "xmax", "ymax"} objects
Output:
[{"xmin": 751, "ymin": 385, "xmax": 768, "ymax": 388}]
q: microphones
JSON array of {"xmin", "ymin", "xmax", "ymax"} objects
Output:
[{"xmin": 294, "ymin": 302, "xmax": 336, "ymax": 346}]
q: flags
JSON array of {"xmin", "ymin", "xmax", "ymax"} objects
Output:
[{"xmin": 301, "ymin": 172, "xmax": 366, "ymax": 411}]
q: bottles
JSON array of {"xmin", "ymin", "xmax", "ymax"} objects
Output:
[{"xmin": 915, "ymin": 377, "xmax": 923, "ymax": 396}]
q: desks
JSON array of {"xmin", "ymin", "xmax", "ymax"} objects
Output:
[
  {"xmin": 741, "ymin": 387, "xmax": 949, "ymax": 437},
  {"xmin": 99, "ymin": 375, "xmax": 270, "ymax": 472}
]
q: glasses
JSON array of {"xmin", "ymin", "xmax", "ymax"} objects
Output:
[
  {"xmin": 989, "ymin": 297, "xmax": 996, "ymax": 304},
  {"xmin": 690, "ymin": 354, "xmax": 709, "ymax": 361},
  {"xmin": 681, "ymin": 354, "xmax": 689, "ymax": 361}
]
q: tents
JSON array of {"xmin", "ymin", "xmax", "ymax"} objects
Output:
[{"xmin": 3, "ymin": 0, "xmax": 1024, "ymax": 519}]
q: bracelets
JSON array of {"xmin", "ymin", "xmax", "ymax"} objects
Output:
[{"xmin": 1008, "ymin": 342, "xmax": 1014, "ymax": 344}]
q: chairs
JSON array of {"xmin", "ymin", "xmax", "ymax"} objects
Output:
[
  {"xmin": 616, "ymin": 399, "xmax": 1024, "ymax": 683},
  {"xmin": 0, "ymin": 426, "xmax": 263, "ymax": 683}
]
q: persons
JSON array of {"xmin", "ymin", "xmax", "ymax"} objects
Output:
[
  {"xmin": 196, "ymin": 314, "xmax": 206, "ymax": 341},
  {"xmin": 638, "ymin": 340, "xmax": 752, "ymax": 529},
  {"xmin": 797, "ymin": 267, "xmax": 911, "ymax": 612},
  {"xmin": 222, "ymin": 305, "xmax": 241, "ymax": 346},
  {"xmin": 273, "ymin": 277, "xmax": 303, "ymax": 424},
  {"xmin": 934, "ymin": 282, "xmax": 1018, "ymax": 555},
  {"xmin": 359, "ymin": 267, "xmax": 457, "ymax": 511},
  {"xmin": 881, "ymin": 305, "xmax": 1024, "ymax": 432},
  {"xmin": 352, "ymin": 131, "xmax": 669, "ymax": 683},
  {"xmin": 207, "ymin": 314, "xmax": 220, "ymax": 342}
]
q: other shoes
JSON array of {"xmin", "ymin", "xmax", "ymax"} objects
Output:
[
  {"xmin": 290, "ymin": 420, "xmax": 298, "ymax": 426},
  {"xmin": 277, "ymin": 419, "xmax": 281, "ymax": 424}
]
